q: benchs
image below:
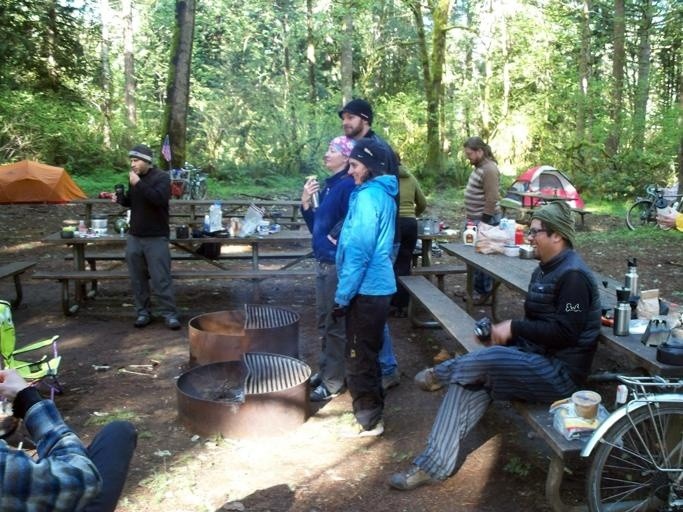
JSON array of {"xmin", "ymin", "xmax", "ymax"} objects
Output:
[
  {"xmin": 63, "ymin": 247, "xmax": 314, "ymax": 299},
  {"xmin": 413, "ymin": 264, "xmax": 467, "ymax": 295},
  {"xmin": 569, "ymin": 208, "xmax": 592, "ymax": 223},
  {"xmin": 397, "ymin": 275, "xmax": 622, "ymax": 511},
  {"xmin": 500, "ymin": 203, "xmax": 529, "ymax": 220},
  {"xmin": 32, "ymin": 264, "xmax": 466, "ymax": 316},
  {"xmin": 0, "ymin": 261, "xmax": 37, "ymax": 307}
]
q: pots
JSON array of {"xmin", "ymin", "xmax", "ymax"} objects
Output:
[{"xmin": 416, "ymin": 218, "xmax": 440, "ymax": 235}]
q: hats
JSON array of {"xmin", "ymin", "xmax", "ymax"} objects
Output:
[
  {"xmin": 128, "ymin": 144, "xmax": 152, "ymax": 163},
  {"xmin": 328, "ymin": 136, "xmax": 355, "ymax": 157},
  {"xmin": 532, "ymin": 201, "xmax": 576, "ymax": 249},
  {"xmin": 350, "ymin": 138, "xmax": 387, "ymax": 176},
  {"xmin": 338, "ymin": 99, "xmax": 372, "ymax": 125}
]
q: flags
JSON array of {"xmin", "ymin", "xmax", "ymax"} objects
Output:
[{"xmin": 162, "ymin": 136, "xmax": 171, "ymax": 162}]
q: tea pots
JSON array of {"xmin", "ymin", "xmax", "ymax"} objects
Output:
[{"xmin": 657, "ymin": 339, "xmax": 683, "ymax": 365}]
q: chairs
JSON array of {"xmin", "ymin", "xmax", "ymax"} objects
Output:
[{"xmin": 0, "ymin": 300, "xmax": 63, "ymax": 397}]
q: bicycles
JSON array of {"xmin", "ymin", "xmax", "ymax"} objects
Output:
[
  {"xmin": 625, "ymin": 184, "xmax": 683, "ymax": 231},
  {"xmin": 180, "ymin": 167, "xmax": 208, "ymax": 200},
  {"xmin": 578, "ymin": 375, "xmax": 683, "ymax": 512}
]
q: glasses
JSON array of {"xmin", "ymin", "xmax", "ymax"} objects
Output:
[{"xmin": 528, "ymin": 228, "xmax": 548, "ymax": 237}]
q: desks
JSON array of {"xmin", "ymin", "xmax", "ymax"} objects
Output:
[
  {"xmin": 439, "ymin": 240, "xmax": 683, "ymax": 379},
  {"xmin": 417, "ymin": 228, "xmax": 461, "ymax": 266},
  {"xmin": 41, "ymin": 227, "xmax": 448, "ymax": 304},
  {"xmin": 508, "ymin": 190, "xmax": 578, "ymax": 211},
  {"xmin": 71, "ymin": 197, "xmax": 303, "ymax": 228}
]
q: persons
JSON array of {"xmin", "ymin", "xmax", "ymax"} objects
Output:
[
  {"xmin": 0, "ymin": 370, "xmax": 137, "ymax": 512},
  {"xmin": 301, "ymin": 136, "xmax": 346, "ymax": 402},
  {"xmin": 388, "ymin": 200, "xmax": 601, "ymax": 491},
  {"xmin": 112, "ymin": 145, "xmax": 182, "ymax": 329},
  {"xmin": 453, "ymin": 137, "xmax": 501, "ymax": 303},
  {"xmin": 391, "ymin": 151, "xmax": 426, "ymax": 319},
  {"xmin": 335, "ymin": 140, "xmax": 399, "ymax": 439},
  {"xmin": 338, "ymin": 100, "xmax": 401, "ymax": 390},
  {"xmin": 656, "ymin": 208, "xmax": 683, "ymax": 233}
]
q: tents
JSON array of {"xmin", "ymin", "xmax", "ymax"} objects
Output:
[
  {"xmin": 0, "ymin": 160, "xmax": 87, "ymax": 202},
  {"xmin": 502, "ymin": 165, "xmax": 585, "ymax": 209}
]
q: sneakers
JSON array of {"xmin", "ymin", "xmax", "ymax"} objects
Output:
[
  {"xmin": 163, "ymin": 316, "xmax": 180, "ymax": 328},
  {"xmin": 339, "ymin": 418, "xmax": 384, "ymax": 437},
  {"xmin": 311, "ymin": 377, "xmax": 347, "ymax": 400},
  {"xmin": 134, "ymin": 315, "xmax": 151, "ymax": 326},
  {"xmin": 389, "ymin": 463, "xmax": 434, "ymax": 490},
  {"xmin": 311, "ymin": 367, "xmax": 323, "ymax": 383},
  {"xmin": 382, "ymin": 371, "xmax": 400, "ymax": 388},
  {"xmin": 415, "ymin": 368, "xmax": 443, "ymax": 392}
]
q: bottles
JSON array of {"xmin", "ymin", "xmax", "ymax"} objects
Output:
[
  {"xmin": 624, "ymin": 258, "xmax": 637, "ymax": 296},
  {"xmin": 418, "ymin": 219, "xmax": 424, "ymax": 234},
  {"xmin": 209, "ymin": 201, "xmax": 223, "ymax": 231},
  {"xmin": 434, "ymin": 220, "xmax": 439, "ymax": 234},
  {"xmin": 515, "ymin": 226, "xmax": 523, "ymax": 245},
  {"xmin": 500, "ymin": 217, "xmax": 508, "ymax": 231},
  {"xmin": 508, "ymin": 218, "xmax": 516, "ymax": 244},
  {"xmin": 613, "ymin": 287, "xmax": 631, "ymax": 336},
  {"xmin": 424, "ymin": 218, "xmax": 430, "ymax": 234}
]
